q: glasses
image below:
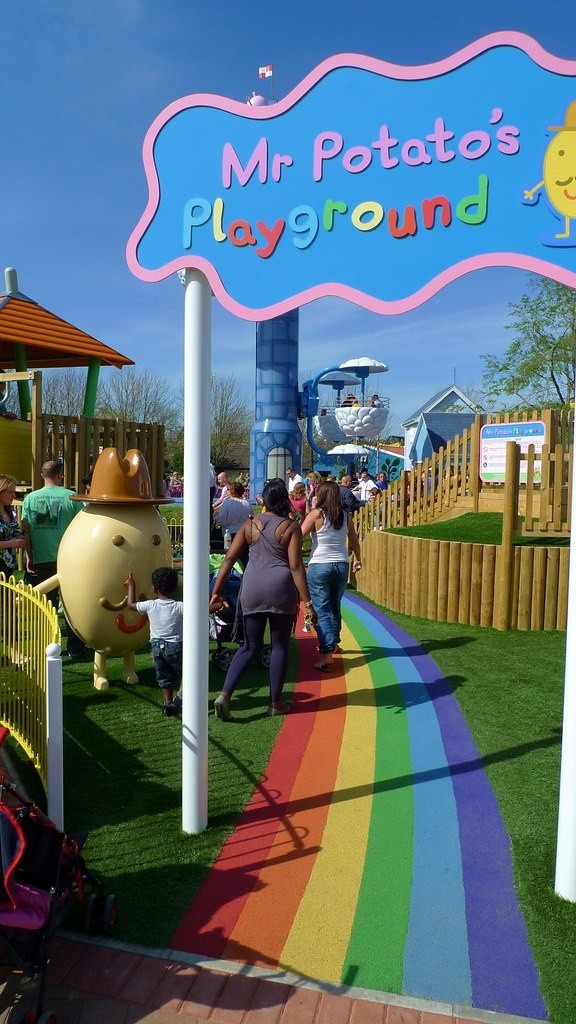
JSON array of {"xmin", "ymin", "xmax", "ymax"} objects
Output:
[{"xmin": 304, "ymin": 614, "xmax": 312, "ymax": 632}]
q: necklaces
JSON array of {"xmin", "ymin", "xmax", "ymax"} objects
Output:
[{"xmin": 3, "ymin": 514, "xmax": 7, "ymax": 517}]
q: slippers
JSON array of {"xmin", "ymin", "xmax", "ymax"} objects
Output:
[{"xmin": 312, "ymin": 662, "xmax": 332, "ymax": 672}]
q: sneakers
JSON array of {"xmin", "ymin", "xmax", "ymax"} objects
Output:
[{"xmin": 163, "ymin": 696, "xmax": 181, "ymax": 715}]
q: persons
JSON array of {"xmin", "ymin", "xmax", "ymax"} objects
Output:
[
  {"xmin": 0, "ymin": 473, "xmax": 34, "ymax": 667},
  {"xmin": 124, "ymin": 568, "xmax": 229, "ymax": 718},
  {"xmin": 207, "ymin": 483, "xmax": 318, "ymax": 718},
  {"xmin": 341, "ymin": 393, "xmax": 385, "ymax": 408},
  {"xmin": 80, "ymin": 473, "xmax": 93, "ymax": 507},
  {"xmin": 20, "ymin": 461, "xmax": 90, "ymax": 658},
  {"xmin": 321, "ymin": 409, "xmax": 327, "ymax": 416},
  {"xmin": 167, "ymin": 464, "xmax": 445, "ymax": 566},
  {"xmin": 302, "ymin": 481, "xmax": 361, "ymax": 674}
]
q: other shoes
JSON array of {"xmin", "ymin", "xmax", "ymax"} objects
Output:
[
  {"xmin": 213, "ymin": 695, "xmax": 232, "ymax": 720},
  {"xmin": 267, "ymin": 702, "xmax": 291, "ymax": 716}
]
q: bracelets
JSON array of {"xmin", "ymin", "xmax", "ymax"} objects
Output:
[{"xmin": 304, "ymin": 600, "xmax": 313, "ymax": 608}]
